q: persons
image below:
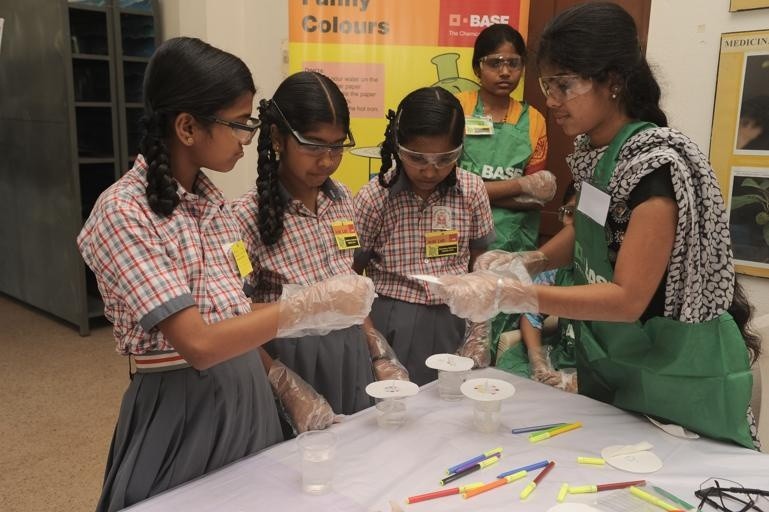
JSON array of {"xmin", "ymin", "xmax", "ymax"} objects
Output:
[
  {"xmin": 351, "ymin": 86, "xmax": 494, "ymax": 390},
  {"xmin": 454, "ymin": 23, "xmax": 548, "ymax": 367},
  {"xmin": 75, "ymin": 35, "xmax": 377, "ymax": 512},
  {"xmin": 440, "ymin": 0, "xmax": 760, "ymax": 450},
  {"xmin": 228, "ymin": 71, "xmax": 409, "ymax": 439}
]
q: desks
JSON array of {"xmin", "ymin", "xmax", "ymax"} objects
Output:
[{"xmin": 120, "ymin": 367, "xmax": 767, "ymax": 512}]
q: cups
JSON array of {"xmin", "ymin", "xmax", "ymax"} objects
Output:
[
  {"xmin": 298, "ymin": 430, "xmax": 342, "ymax": 496},
  {"xmin": 437, "ymin": 371, "xmax": 469, "ymax": 402},
  {"xmin": 473, "ymin": 401, "xmax": 502, "ymax": 434},
  {"xmin": 374, "ymin": 397, "xmax": 408, "ymax": 434}
]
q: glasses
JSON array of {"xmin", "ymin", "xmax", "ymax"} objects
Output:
[
  {"xmin": 694, "ymin": 477, "xmax": 768, "ymax": 512},
  {"xmin": 270, "ymin": 97, "xmax": 355, "ymax": 155},
  {"xmin": 538, "ymin": 73, "xmax": 593, "ymax": 103},
  {"xmin": 198, "ymin": 114, "xmax": 262, "ymax": 142},
  {"xmin": 392, "ymin": 118, "xmax": 463, "ymax": 169},
  {"xmin": 478, "ymin": 54, "xmax": 524, "ymax": 71}
]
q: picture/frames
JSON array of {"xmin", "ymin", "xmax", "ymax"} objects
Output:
[
  {"xmin": 729, "ymin": 0, "xmax": 768, "ymax": 11},
  {"xmin": 708, "ymin": 29, "xmax": 769, "ymax": 278}
]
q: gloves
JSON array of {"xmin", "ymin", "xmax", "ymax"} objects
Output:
[
  {"xmin": 519, "ymin": 171, "xmax": 558, "ymax": 200},
  {"xmin": 456, "ymin": 322, "xmax": 492, "ymax": 370},
  {"xmin": 541, "ymin": 368, "xmax": 577, "ymax": 394},
  {"xmin": 365, "ymin": 328, "xmax": 409, "ymax": 382},
  {"xmin": 275, "ymin": 274, "xmax": 374, "ymax": 337},
  {"xmin": 473, "ymin": 250, "xmax": 546, "ymax": 275},
  {"xmin": 268, "ymin": 359, "xmax": 344, "ymax": 434},
  {"xmin": 436, "ymin": 271, "xmax": 539, "ymax": 322},
  {"xmin": 528, "ymin": 346, "xmax": 561, "ymax": 385}
]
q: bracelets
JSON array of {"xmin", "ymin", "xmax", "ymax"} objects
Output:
[{"xmin": 494, "ymin": 274, "xmax": 504, "ymax": 312}]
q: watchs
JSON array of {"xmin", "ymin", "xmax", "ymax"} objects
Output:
[{"xmin": 370, "ymin": 349, "xmax": 393, "ymax": 363}]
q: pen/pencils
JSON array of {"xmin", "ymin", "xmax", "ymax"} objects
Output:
[
  {"xmin": 456, "ymin": 451, "xmax": 500, "ymax": 473},
  {"xmin": 408, "ymin": 480, "xmax": 484, "ymax": 504},
  {"xmin": 512, "ymin": 422, "xmax": 566, "ymax": 434},
  {"xmin": 568, "ymin": 479, "xmax": 646, "ymax": 497},
  {"xmin": 520, "ymin": 461, "xmax": 556, "ymax": 500},
  {"xmin": 530, "ymin": 424, "xmax": 568, "ymax": 436},
  {"xmin": 530, "ymin": 422, "xmax": 583, "ymax": 442},
  {"xmin": 496, "ymin": 461, "xmax": 548, "ymax": 480},
  {"xmin": 464, "ymin": 469, "xmax": 528, "ymax": 502},
  {"xmin": 441, "ymin": 456, "xmax": 499, "ymax": 490},
  {"xmin": 630, "ymin": 485, "xmax": 684, "ymax": 512},
  {"xmin": 448, "ymin": 446, "xmax": 502, "ymax": 471},
  {"xmin": 653, "ymin": 486, "xmax": 697, "ymax": 512}
]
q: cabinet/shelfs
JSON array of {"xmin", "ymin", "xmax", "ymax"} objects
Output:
[{"xmin": 0, "ymin": 0, "xmax": 164, "ymax": 336}]
